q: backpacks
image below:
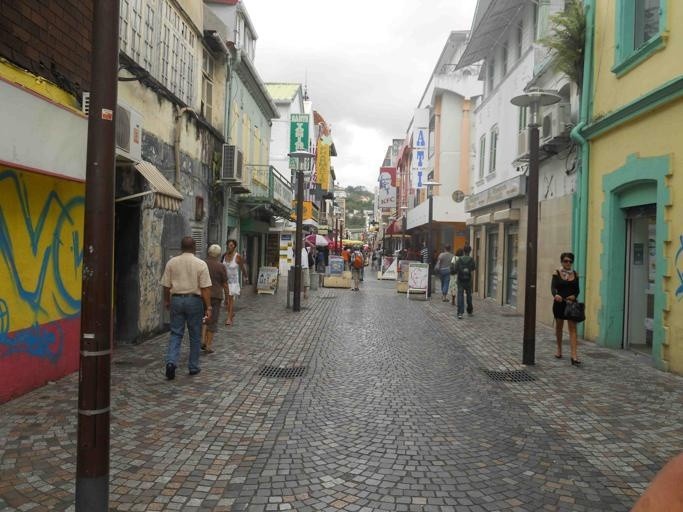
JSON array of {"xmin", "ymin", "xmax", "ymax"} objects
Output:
[{"xmin": 460, "ymin": 257, "xmax": 473, "ymax": 281}]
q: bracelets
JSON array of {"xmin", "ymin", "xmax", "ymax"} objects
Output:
[
  {"xmin": 206, "ymin": 306, "xmax": 212, "ymax": 310},
  {"xmin": 244, "ymin": 277, "xmax": 248, "ymax": 281}
]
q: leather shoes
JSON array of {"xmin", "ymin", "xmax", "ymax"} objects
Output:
[
  {"xmin": 190, "ymin": 368, "xmax": 200, "ymax": 374},
  {"xmin": 165, "ymin": 362, "xmax": 177, "ymax": 378}
]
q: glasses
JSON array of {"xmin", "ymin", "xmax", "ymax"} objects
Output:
[{"xmin": 563, "ymin": 259, "xmax": 574, "ymax": 264}]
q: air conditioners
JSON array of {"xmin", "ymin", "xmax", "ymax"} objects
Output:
[
  {"xmin": 221, "ymin": 144, "xmax": 244, "ymax": 185},
  {"xmin": 82, "ymin": 92, "xmax": 143, "ymax": 163},
  {"xmin": 540, "ymin": 103, "xmax": 571, "ymax": 146}
]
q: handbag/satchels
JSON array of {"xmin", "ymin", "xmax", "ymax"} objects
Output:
[
  {"xmin": 308, "ymin": 252, "xmax": 314, "ymax": 266},
  {"xmin": 450, "ymin": 262, "xmax": 457, "ymax": 275},
  {"xmin": 565, "ymin": 302, "xmax": 585, "ymax": 322},
  {"xmin": 353, "ymin": 256, "xmax": 362, "ymax": 270}
]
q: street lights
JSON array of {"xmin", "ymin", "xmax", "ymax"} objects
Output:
[
  {"xmin": 422, "ymin": 179, "xmax": 442, "ymax": 298},
  {"xmin": 333, "ymin": 206, "xmax": 339, "ymax": 256},
  {"xmin": 287, "ymin": 148, "xmax": 317, "ymax": 312},
  {"xmin": 337, "ymin": 218, "xmax": 344, "ymax": 256},
  {"xmin": 398, "ymin": 205, "xmax": 408, "ymax": 260},
  {"xmin": 509, "ymin": 86, "xmax": 561, "ymax": 367},
  {"xmin": 381, "ymin": 222, "xmax": 387, "ymax": 257},
  {"xmin": 369, "ymin": 230, "xmax": 377, "ymax": 250},
  {"xmin": 346, "ymin": 230, "xmax": 353, "ymax": 241}
]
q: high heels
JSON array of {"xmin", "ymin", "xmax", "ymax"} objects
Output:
[{"xmin": 571, "ymin": 357, "xmax": 581, "ymax": 365}]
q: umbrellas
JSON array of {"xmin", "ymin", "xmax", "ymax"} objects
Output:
[{"xmin": 303, "ymin": 234, "xmax": 331, "ymax": 259}]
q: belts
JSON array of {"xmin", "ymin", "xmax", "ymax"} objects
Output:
[{"xmin": 172, "ymin": 293, "xmax": 201, "ymax": 297}]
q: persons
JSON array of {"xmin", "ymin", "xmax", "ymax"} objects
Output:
[
  {"xmin": 551, "ymin": 252, "xmax": 584, "ymax": 365},
  {"xmin": 201, "ymin": 244, "xmax": 230, "ymax": 354},
  {"xmin": 220, "ymin": 239, "xmax": 249, "ymax": 326},
  {"xmin": 379, "ymin": 172, "xmax": 396, "ymax": 207},
  {"xmin": 294, "ymin": 239, "xmax": 477, "ymax": 319},
  {"xmin": 159, "ymin": 236, "xmax": 213, "ymax": 379}
]
